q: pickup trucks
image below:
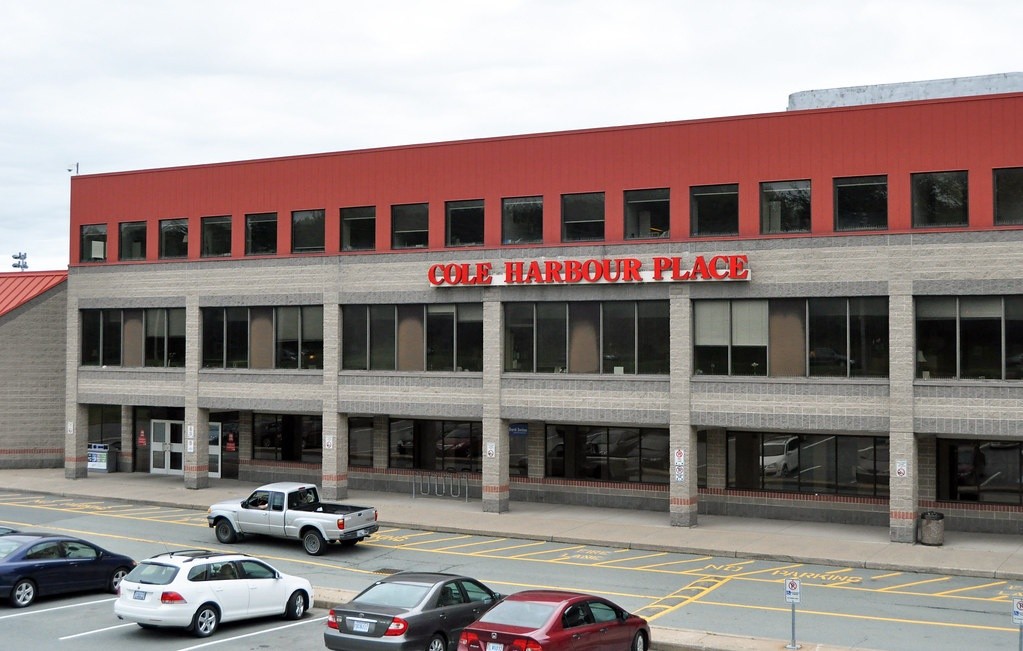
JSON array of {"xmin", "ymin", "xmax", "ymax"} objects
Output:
[{"xmin": 207, "ymin": 481, "xmax": 380, "ymax": 557}]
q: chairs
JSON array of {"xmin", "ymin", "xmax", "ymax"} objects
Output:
[
  {"xmin": 437, "ymin": 586, "xmax": 457, "ymax": 607},
  {"xmin": 562, "ymin": 607, "xmax": 583, "ymax": 627},
  {"xmin": 215, "ymin": 564, "xmax": 236, "ymax": 579}
]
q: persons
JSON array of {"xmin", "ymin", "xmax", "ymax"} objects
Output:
[{"xmin": 258, "ymin": 503, "xmax": 269, "ymax": 509}]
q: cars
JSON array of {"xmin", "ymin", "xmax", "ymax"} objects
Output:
[
  {"xmin": 855, "ymin": 444, "xmax": 891, "ymax": 493},
  {"xmin": 633, "ymin": 432, "xmax": 670, "ymax": 479},
  {"xmin": 586, "ymin": 431, "xmax": 636, "ymax": 471},
  {"xmin": 0, "ymin": 531, "xmax": 138, "ymax": 610},
  {"xmin": 114, "ymin": 548, "xmax": 315, "ymax": 638},
  {"xmin": 758, "ymin": 437, "xmax": 800, "ymax": 480},
  {"xmin": 439, "ymin": 425, "xmax": 482, "ymax": 459},
  {"xmin": 510, "ymin": 427, "xmax": 563, "ymax": 471},
  {"xmin": 396, "ymin": 429, "xmax": 416, "ymax": 456},
  {"xmin": 324, "ymin": 572, "xmax": 511, "ymax": 651},
  {"xmin": 458, "ymin": 590, "xmax": 657, "ymax": 651},
  {"xmin": 951, "ymin": 445, "xmax": 982, "ymax": 497},
  {"xmin": 257, "ymin": 417, "xmax": 322, "ymax": 452}
]
